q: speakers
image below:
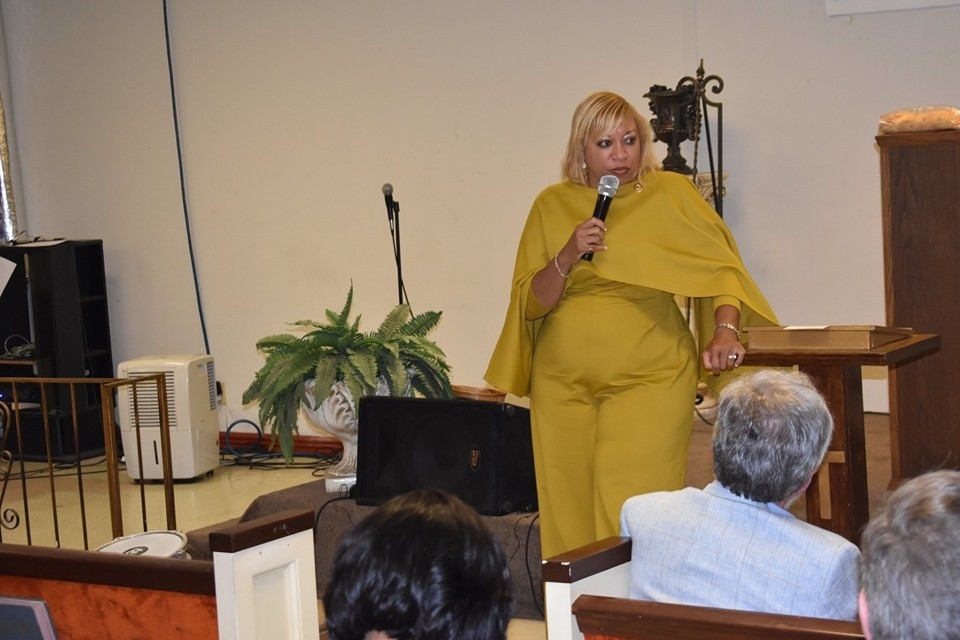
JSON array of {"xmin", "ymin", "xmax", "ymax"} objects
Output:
[
  {"xmin": 355, "ymin": 396, "xmax": 536, "ymax": 520},
  {"xmin": 117, "ymin": 354, "xmax": 221, "ymax": 483},
  {"xmin": 0, "ymin": 237, "xmax": 116, "ymax": 464}
]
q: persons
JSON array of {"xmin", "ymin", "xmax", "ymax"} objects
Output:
[
  {"xmin": 482, "ymin": 93, "xmax": 781, "ymax": 639},
  {"xmin": 323, "ymin": 489, "xmax": 515, "ymax": 640},
  {"xmin": 856, "ymin": 469, "xmax": 960, "ymax": 640},
  {"xmin": 620, "ymin": 369, "xmax": 862, "ymax": 622}
]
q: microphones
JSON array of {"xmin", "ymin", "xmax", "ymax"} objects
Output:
[
  {"xmin": 381, "ymin": 183, "xmax": 394, "ymax": 235},
  {"xmin": 581, "ymin": 175, "xmax": 619, "ymax": 261}
]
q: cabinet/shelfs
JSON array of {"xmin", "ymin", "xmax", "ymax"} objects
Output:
[{"xmin": 0, "ymin": 239, "xmax": 114, "ymax": 466}]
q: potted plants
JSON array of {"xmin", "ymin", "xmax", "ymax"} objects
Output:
[{"xmin": 242, "ymin": 276, "xmax": 454, "ymax": 493}]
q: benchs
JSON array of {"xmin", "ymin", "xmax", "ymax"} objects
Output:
[{"xmin": 1, "ymin": 509, "xmax": 319, "ymax": 640}]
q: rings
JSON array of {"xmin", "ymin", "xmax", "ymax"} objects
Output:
[{"xmin": 728, "ymin": 355, "xmax": 737, "ymax": 359}]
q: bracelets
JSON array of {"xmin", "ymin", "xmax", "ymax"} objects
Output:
[
  {"xmin": 715, "ymin": 323, "xmax": 740, "ymax": 341},
  {"xmin": 554, "ymin": 254, "xmax": 569, "ymax": 278}
]
